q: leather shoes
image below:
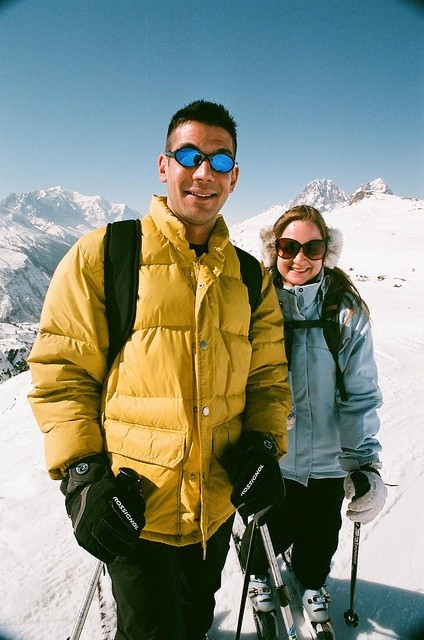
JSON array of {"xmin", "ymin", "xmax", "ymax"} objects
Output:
[
  {"xmin": 342, "ymin": 465, "xmax": 388, "ymax": 524},
  {"xmin": 224, "ymin": 432, "xmax": 287, "ymax": 526},
  {"xmin": 58, "ymin": 453, "xmax": 146, "ymax": 564}
]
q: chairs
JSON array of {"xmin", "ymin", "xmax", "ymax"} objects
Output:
[
  {"xmin": 247, "ymin": 576, "xmax": 275, "ymax": 613},
  {"xmin": 301, "ymin": 587, "xmax": 329, "ymax": 623}
]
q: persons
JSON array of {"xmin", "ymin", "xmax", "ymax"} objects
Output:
[
  {"xmin": 238, "ymin": 205, "xmax": 387, "ymax": 625},
  {"xmin": 28, "ymin": 101, "xmax": 294, "ymax": 640}
]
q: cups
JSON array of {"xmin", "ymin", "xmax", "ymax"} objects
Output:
[
  {"xmin": 274, "ymin": 238, "xmax": 327, "ymax": 261},
  {"xmin": 164, "ymin": 145, "xmax": 236, "ymax": 174}
]
